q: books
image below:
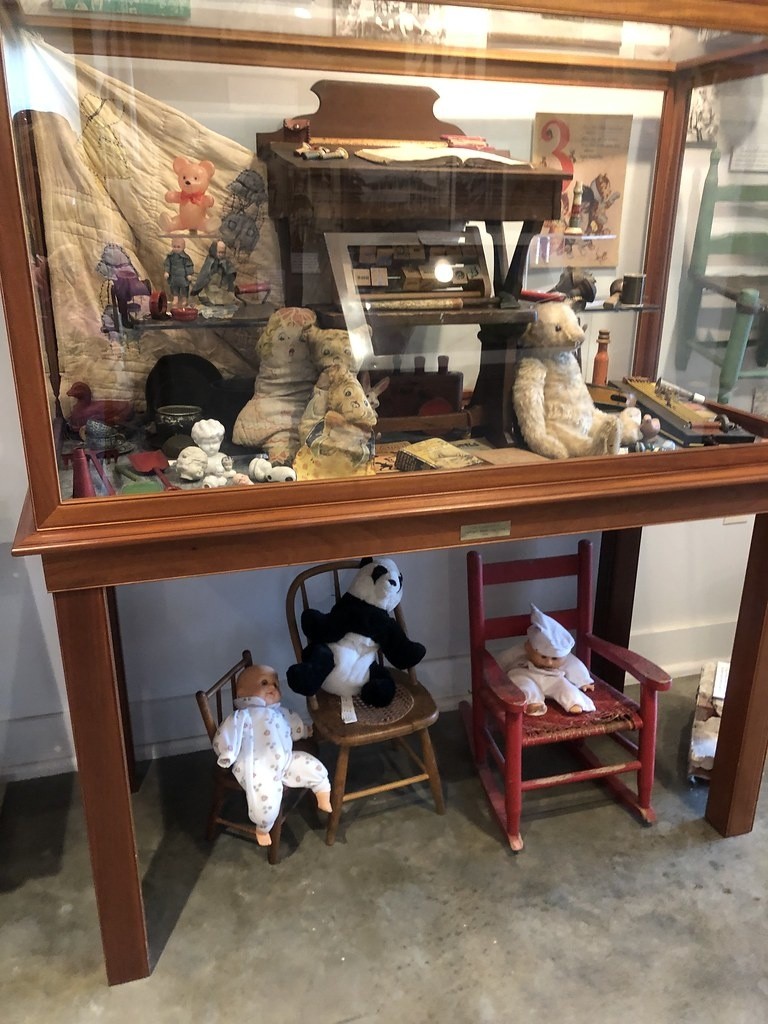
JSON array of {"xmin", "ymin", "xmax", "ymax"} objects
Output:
[{"xmin": 356, "ymin": 146, "xmax": 535, "ymax": 170}]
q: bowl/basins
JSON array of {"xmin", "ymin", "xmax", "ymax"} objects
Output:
[
  {"xmin": 200, "ymin": 305, "xmax": 238, "ymax": 319},
  {"xmin": 170, "ymin": 308, "xmax": 198, "ymax": 320}
]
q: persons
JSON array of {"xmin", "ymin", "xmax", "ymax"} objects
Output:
[
  {"xmin": 210, "ymin": 664, "xmax": 333, "ymax": 846},
  {"xmin": 163, "ymin": 238, "xmax": 195, "ymax": 310},
  {"xmin": 496, "ymin": 603, "xmax": 597, "ymax": 715},
  {"xmin": 176, "ymin": 446, "xmax": 208, "ymax": 480},
  {"xmin": 189, "ymin": 239, "xmax": 238, "ymax": 306},
  {"xmin": 191, "ymin": 419, "xmax": 228, "ymax": 474}
]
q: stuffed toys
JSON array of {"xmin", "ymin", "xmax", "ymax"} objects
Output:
[
  {"xmin": 286, "ymin": 556, "xmax": 427, "ymax": 708},
  {"xmin": 513, "ymin": 301, "xmax": 644, "ymax": 460},
  {"xmin": 230, "ymin": 307, "xmax": 321, "ymax": 466},
  {"xmin": 293, "ymin": 324, "xmax": 389, "ymax": 482}
]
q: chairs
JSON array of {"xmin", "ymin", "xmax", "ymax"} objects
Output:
[
  {"xmin": 468, "ymin": 539, "xmax": 672, "ymax": 853},
  {"xmin": 286, "ymin": 560, "xmax": 444, "ymax": 846},
  {"xmin": 669, "ymin": 148, "xmax": 768, "ymax": 406},
  {"xmin": 195, "ymin": 650, "xmax": 319, "ymax": 865}
]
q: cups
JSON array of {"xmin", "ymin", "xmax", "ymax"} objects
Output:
[
  {"xmin": 85, "ymin": 420, "xmax": 126, "ymax": 449},
  {"xmin": 157, "ymin": 405, "xmax": 202, "ymax": 460},
  {"xmin": 622, "ymin": 274, "xmax": 643, "ymax": 304}
]
